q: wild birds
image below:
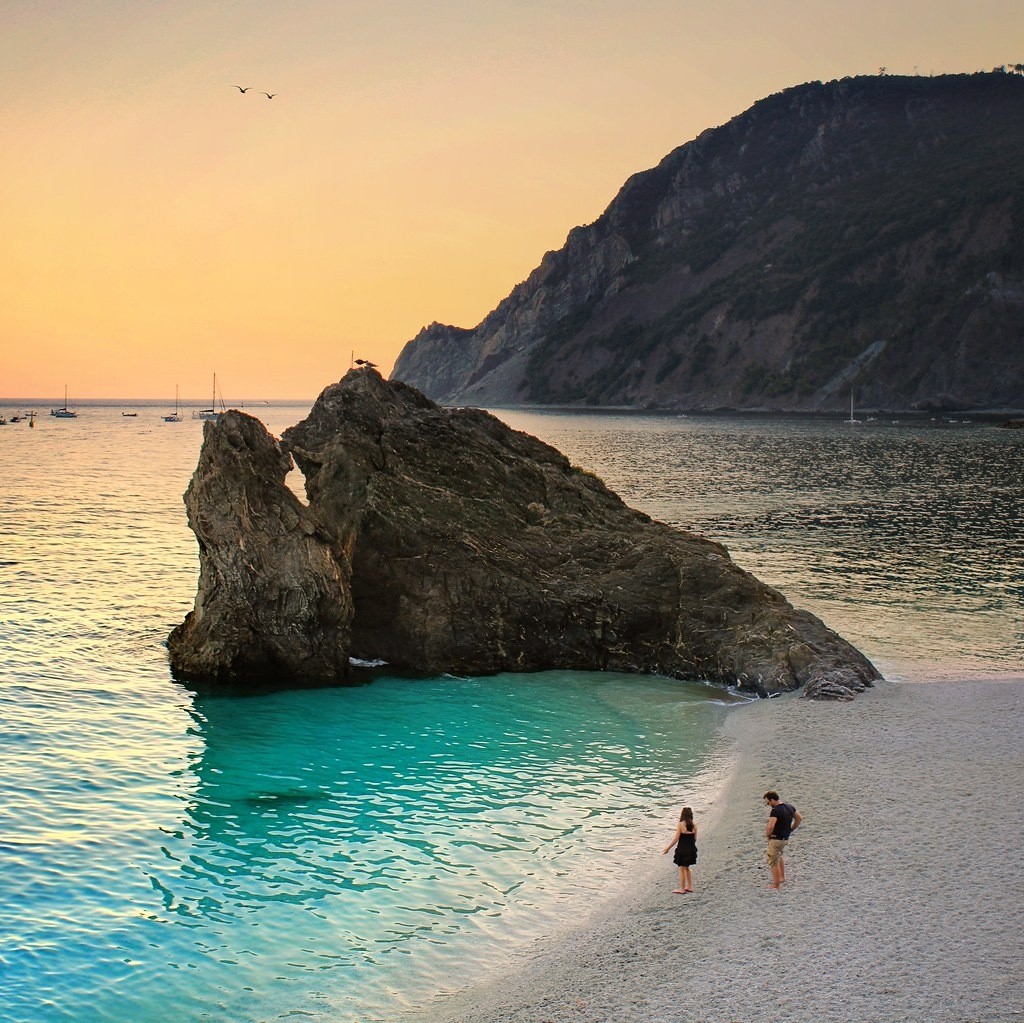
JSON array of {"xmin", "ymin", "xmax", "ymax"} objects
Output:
[
  {"xmin": 262, "ymin": 92, "xmax": 278, "ymax": 100},
  {"xmin": 232, "ymin": 84, "xmax": 252, "ymax": 93}
]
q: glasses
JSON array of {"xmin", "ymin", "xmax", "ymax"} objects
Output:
[{"xmin": 767, "ymin": 801, "xmax": 770, "ymax": 805}]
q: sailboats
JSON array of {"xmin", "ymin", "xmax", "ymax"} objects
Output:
[{"xmin": 161, "ymin": 372, "xmax": 220, "ymax": 422}]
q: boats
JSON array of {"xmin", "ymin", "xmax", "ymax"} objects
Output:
[
  {"xmin": 843, "ymin": 385, "xmax": 974, "ymax": 425},
  {"xmin": 122, "ymin": 413, "xmax": 137, "ymax": 417},
  {"xmin": 0, "ymin": 384, "xmax": 79, "ymax": 426}
]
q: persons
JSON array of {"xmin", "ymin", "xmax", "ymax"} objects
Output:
[
  {"xmin": 662, "ymin": 807, "xmax": 699, "ymax": 894},
  {"xmin": 763, "ymin": 791, "xmax": 802, "ymax": 886}
]
